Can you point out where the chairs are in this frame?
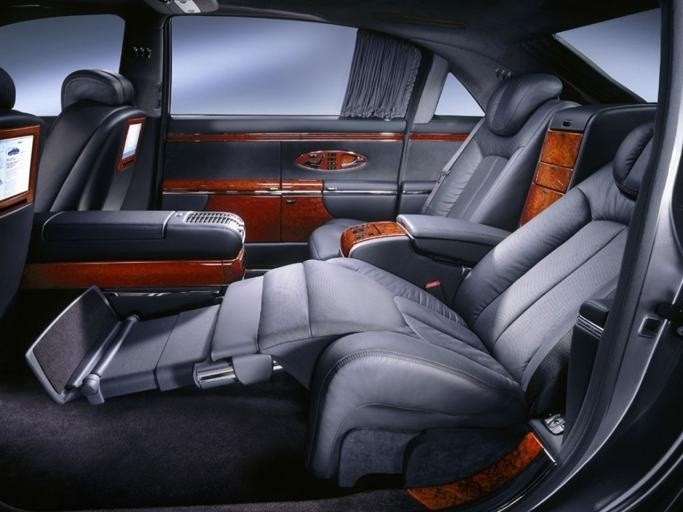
[301,70,585,263]
[21,115,658,486]
[33,66,151,214]
[2,69,46,313]
